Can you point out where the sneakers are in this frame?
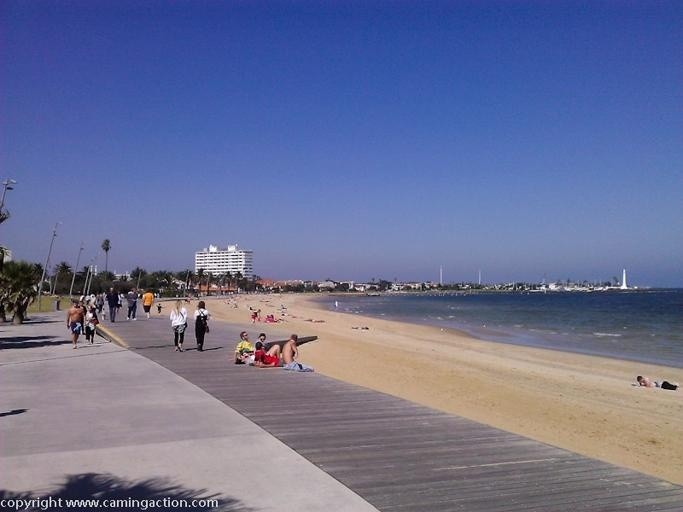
[176,344,184,352]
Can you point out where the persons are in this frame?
[630,374,679,391]
[255,332,280,359]
[169,300,189,351]
[255,341,281,368]
[282,334,314,372]
[223,295,287,324]
[235,353,256,364]
[194,300,209,352]
[66,286,163,350]
[233,331,254,363]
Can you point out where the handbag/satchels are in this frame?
[206,325,209,334]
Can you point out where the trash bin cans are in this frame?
[53,300,61,310]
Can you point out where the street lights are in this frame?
[38,221,63,311]
[70,240,98,296]
[1,178,17,210]
